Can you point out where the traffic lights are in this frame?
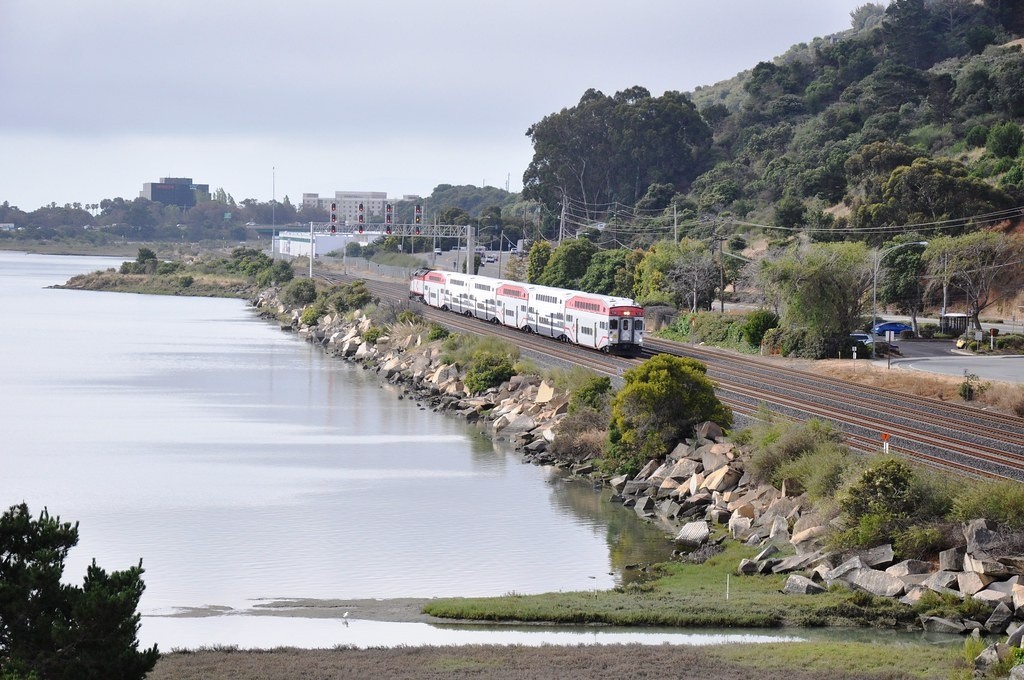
[358,214,364,224]
[358,224,363,233]
[331,213,336,223]
[415,226,421,235]
[414,213,421,224]
[385,203,391,213]
[414,205,422,214]
[385,214,391,223]
[331,202,336,211]
[386,225,392,234]
[331,224,336,234]
[358,203,364,211]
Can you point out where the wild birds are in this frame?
[343,612,349,620]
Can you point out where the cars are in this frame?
[475,244,498,267]
[510,248,518,255]
[434,247,442,255]
[849,333,873,345]
[869,322,913,337]
[866,341,901,358]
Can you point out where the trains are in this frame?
[408,269,646,359]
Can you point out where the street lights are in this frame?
[870,242,929,358]
[477,216,499,254]
